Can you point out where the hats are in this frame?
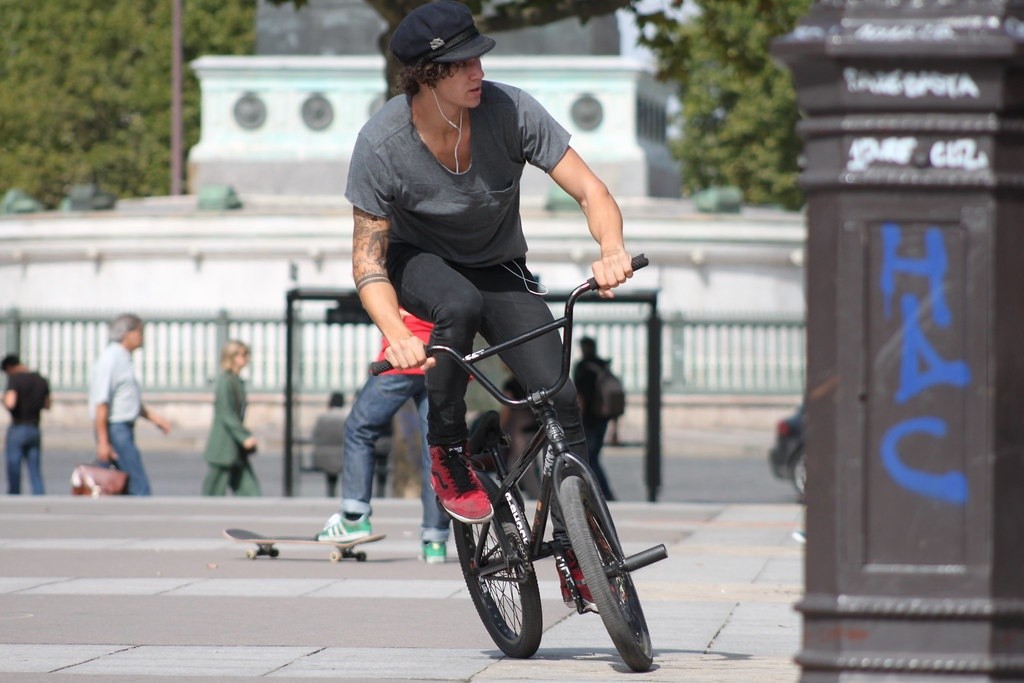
[390,1,497,62]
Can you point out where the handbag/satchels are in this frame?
[70,455,129,497]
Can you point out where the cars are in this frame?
[769,402,805,505]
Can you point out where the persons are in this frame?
[318,304,467,566]
[311,388,352,498]
[84,313,171,496]
[0,351,55,495]
[369,418,394,499]
[194,339,268,496]
[307,1,642,525]
[564,334,627,502]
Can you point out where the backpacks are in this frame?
[584,360,625,419]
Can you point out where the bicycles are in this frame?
[369,253,669,672]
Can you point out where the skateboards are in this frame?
[224,528,388,562]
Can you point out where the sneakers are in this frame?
[418,541,446,564]
[556,550,619,613]
[314,513,372,543]
[429,440,495,524]
